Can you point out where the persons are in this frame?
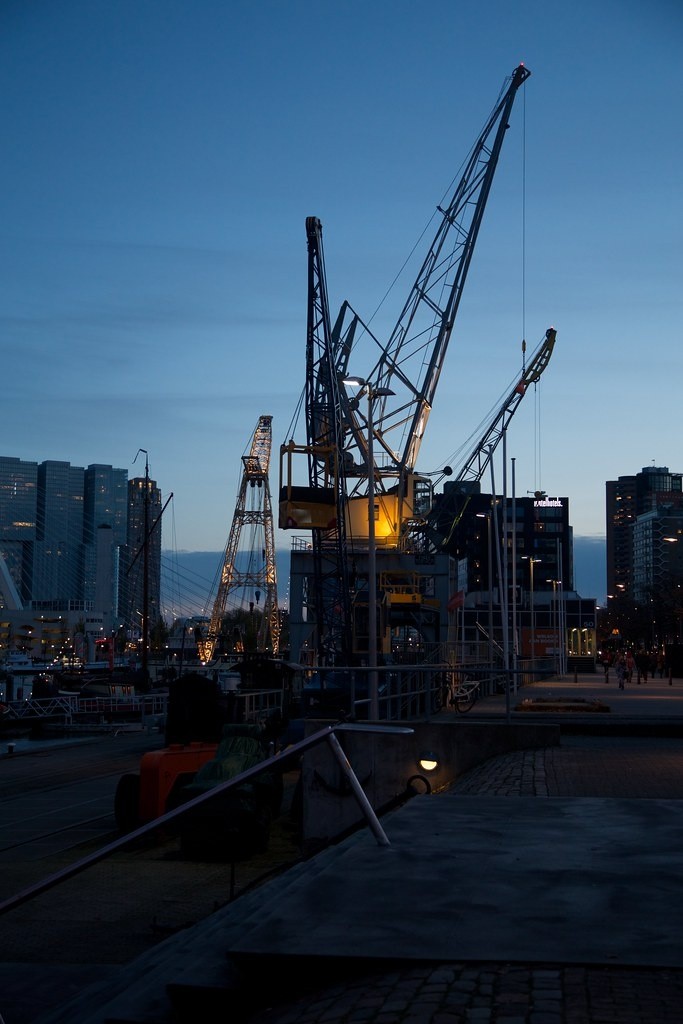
[600,648,665,691]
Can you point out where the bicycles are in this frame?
[432,658,481,712]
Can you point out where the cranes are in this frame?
[182,61,562,712]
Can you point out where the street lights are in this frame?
[546,579,562,660]
[520,555,543,658]
